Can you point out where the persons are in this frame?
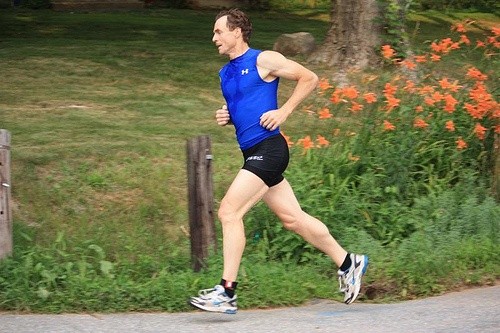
[187,7,369,314]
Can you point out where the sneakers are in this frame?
[187,283,237,314]
[337,252,368,304]
